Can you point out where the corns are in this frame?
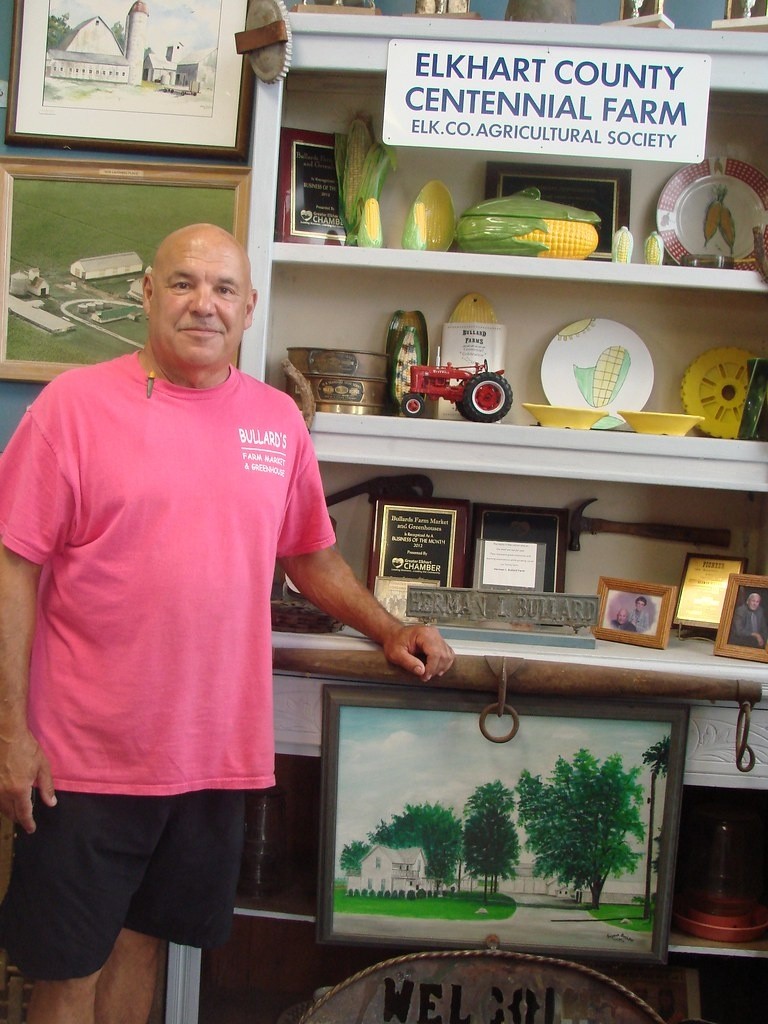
[390,326,422,417]
[332,119,665,266]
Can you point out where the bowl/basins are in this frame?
[617,409,705,436]
[522,403,609,430]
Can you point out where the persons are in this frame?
[628,596,650,632]
[0,220,454,1023]
[730,593,768,649]
[611,608,636,631]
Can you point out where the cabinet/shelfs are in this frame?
[162,12,768,1024]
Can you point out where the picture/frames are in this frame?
[374,576,440,626]
[713,573,768,663]
[1,155,265,384]
[589,576,679,649]
[484,161,631,261]
[4,0,254,160]
[318,685,688,967]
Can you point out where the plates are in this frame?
[540,318,655,429]
[656,154,768,271]
[384,309,430,417]
[416,179,456,252]
